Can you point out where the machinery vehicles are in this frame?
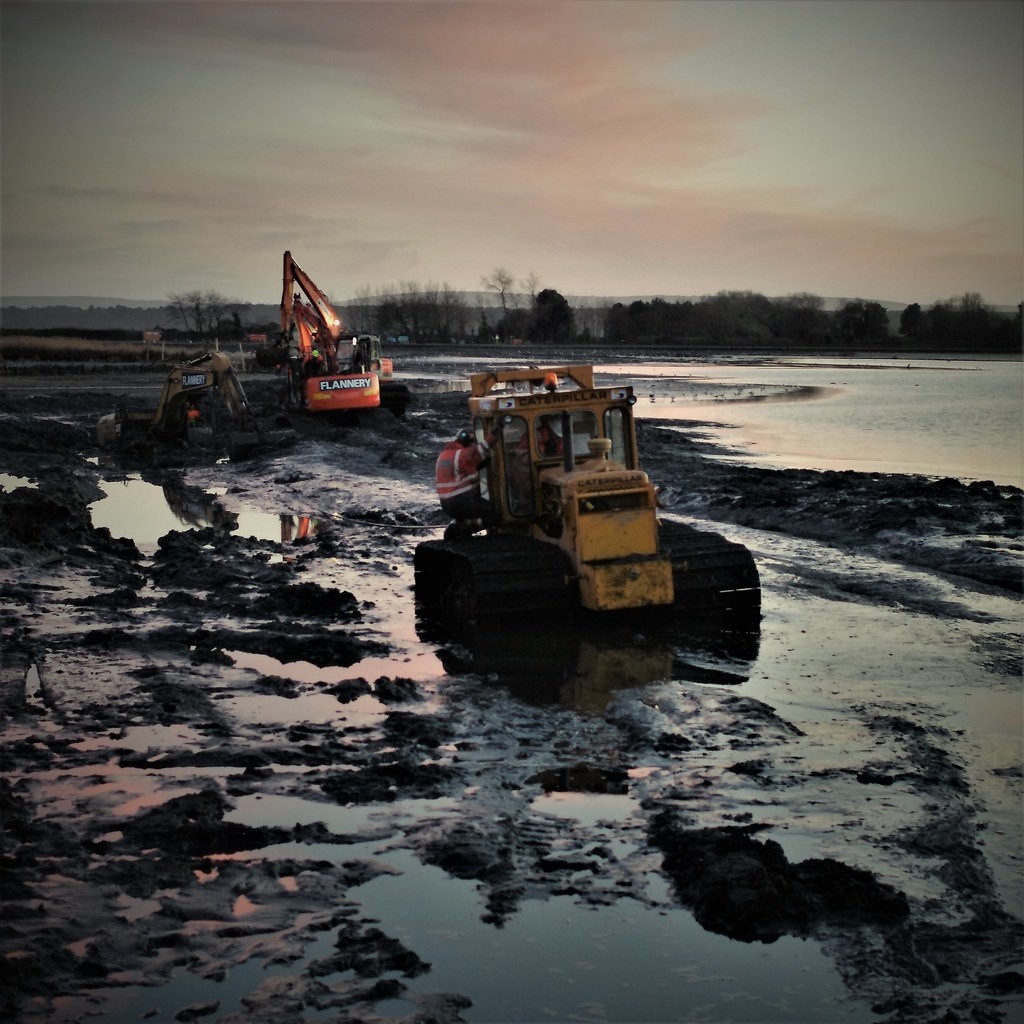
[96,351,279,466]
[255,250,411,440]
[413,364,762,706]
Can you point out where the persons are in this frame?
[295,351,306,407]
[436,424,503,534]
[495,334,499,345]
[188,404,205,427]
[312,349,325,377]
[510,418,564,513]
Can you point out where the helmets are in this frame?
[313,351,317,357]
[458,430,473,442]
